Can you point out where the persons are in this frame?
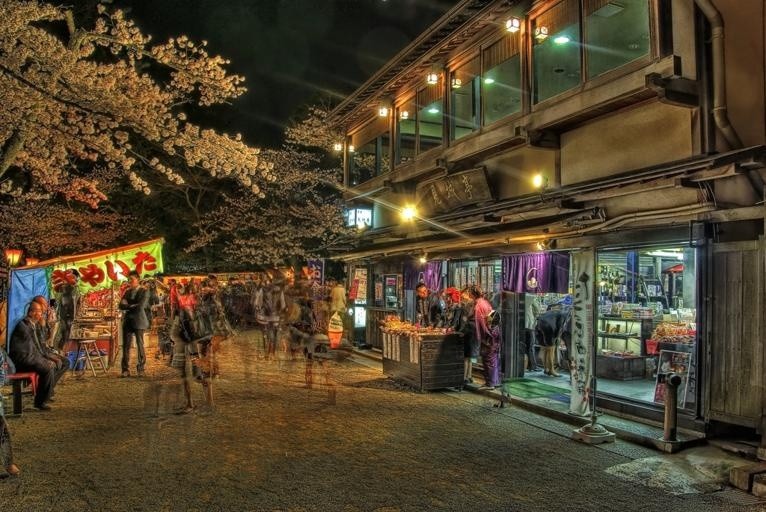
[0,412,20,477]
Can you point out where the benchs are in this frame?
[5,373,38,414]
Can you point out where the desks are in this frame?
[378,326,465,389]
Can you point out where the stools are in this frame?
[73,339,107,376]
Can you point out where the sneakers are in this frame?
[34,396,55,412]
[137,370,145,377]
[120,369,130,377]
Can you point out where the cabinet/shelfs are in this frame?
[641,274,671,315]
[596,316,653,380]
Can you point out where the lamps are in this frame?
[334,141,342,150]
[401,111,408,119]
[379,108,388,117]
[451,78,462,88]
[534,25,548,39]
[505,12,520,33]
[426,73,438,84]
[349,145,354,152]
[532,175,550,202]
